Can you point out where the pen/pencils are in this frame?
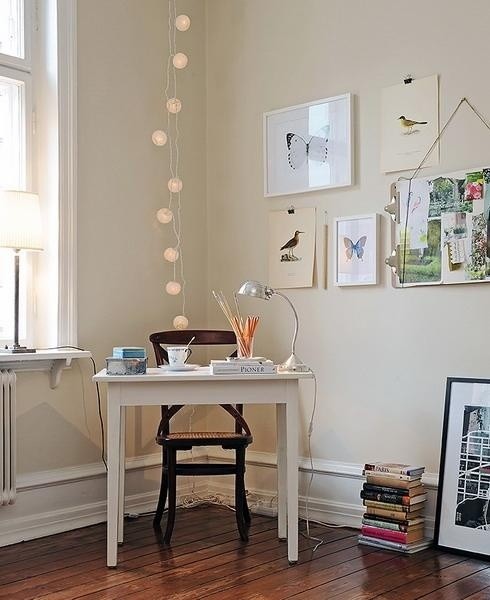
[231,315,259,358]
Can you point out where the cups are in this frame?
[237,337,254,359]
[167,347,192,367]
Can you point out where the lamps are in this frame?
[150,0,190,332]
[0,190,47,354]
[237,278,312,373]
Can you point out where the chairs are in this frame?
[149,327,255,546]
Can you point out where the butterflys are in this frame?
[344,236,367,262]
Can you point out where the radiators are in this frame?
[1,367,19,508]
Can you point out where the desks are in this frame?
[91,367,317,571]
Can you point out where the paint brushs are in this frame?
[212,289,250,358]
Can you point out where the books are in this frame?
[359,463,434,554]
[209,360,278,374]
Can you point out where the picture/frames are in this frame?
[260,92,355,198]
[430,376,490,563]
[332,212,383,288]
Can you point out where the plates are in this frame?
[160,365,199,371]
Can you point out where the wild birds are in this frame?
[279,230,305,260]
[397,116,428,135]
